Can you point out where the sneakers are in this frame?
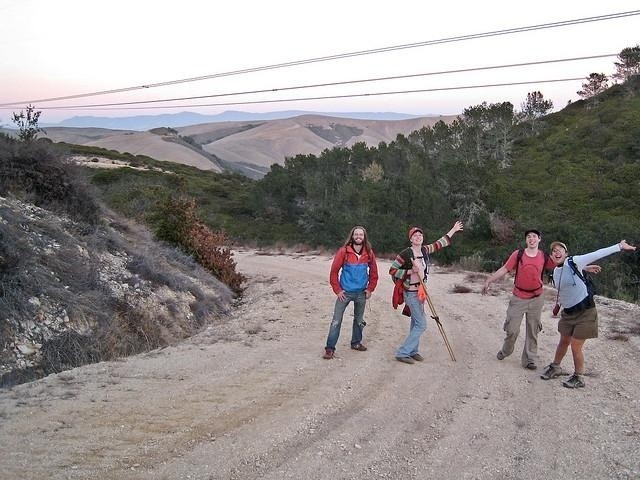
[540,362,563,381]
[563,372,585,388]
[496,349,509,360]
[394,356,416,365]
[323,345,336,360]
[350,343,368,351]
[411,353,425,361]
[526,360,537,370]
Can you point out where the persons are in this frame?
[540,240,636,388]
[323,226,378,359]
[389,221,463,363]
[481,229,602,368]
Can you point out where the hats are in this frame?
[408,226,424,239]
[524,228,542,237]
[550,240,568,253]
